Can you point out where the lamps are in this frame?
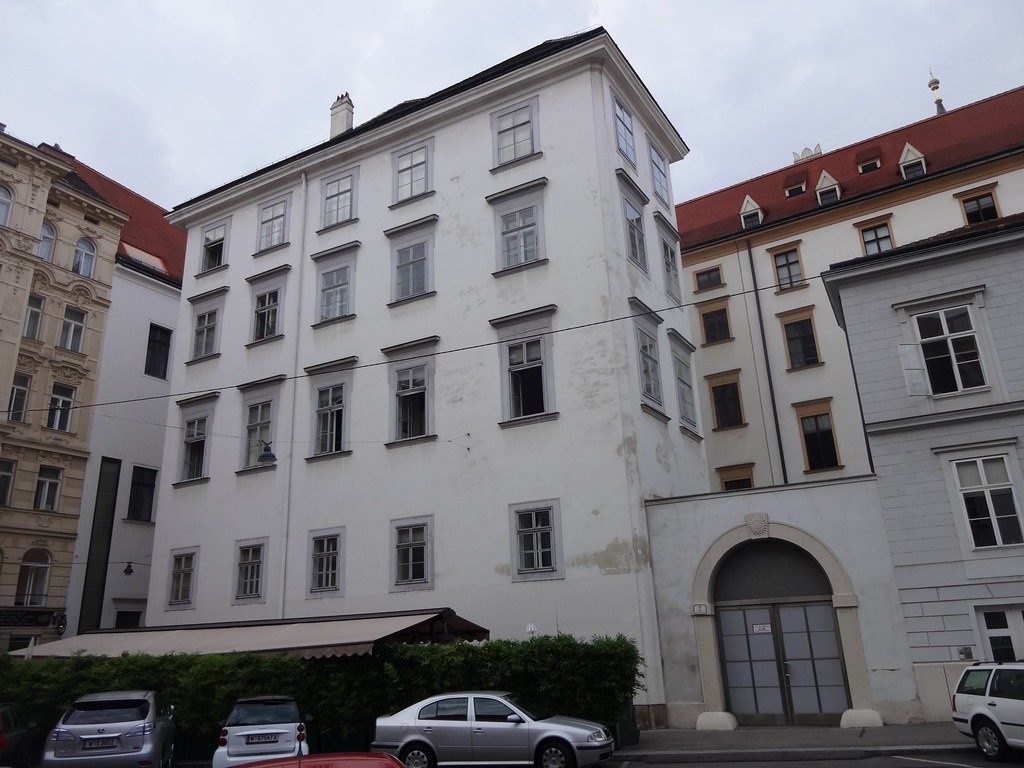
[256,442,278,466]
[526,622,536,638]
[122,563,134,575]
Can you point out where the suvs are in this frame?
[952,660,1024,762]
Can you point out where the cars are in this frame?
[370,690,616,768]
[39,689,176,768]
[227,751,405,768]
[212,696,309,768]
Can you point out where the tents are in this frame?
[5,605,490,660]
